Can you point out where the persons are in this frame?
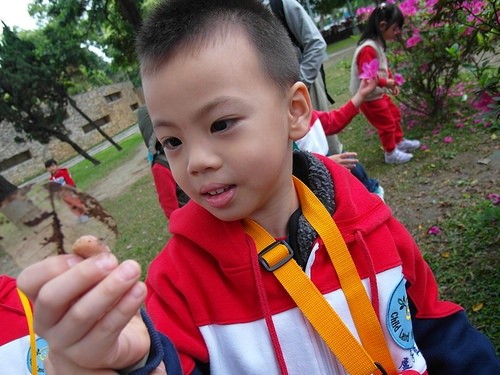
[45,160,76,187]
[1,274,49,374]
[17,0,500,375]
[136,105,191,220]
[295,60,385,203]
[348,4,421,164]
[262,0,344,157]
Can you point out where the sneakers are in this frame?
[395,139,420,152]
[374,185,384,202]
[384,149,413,164]
